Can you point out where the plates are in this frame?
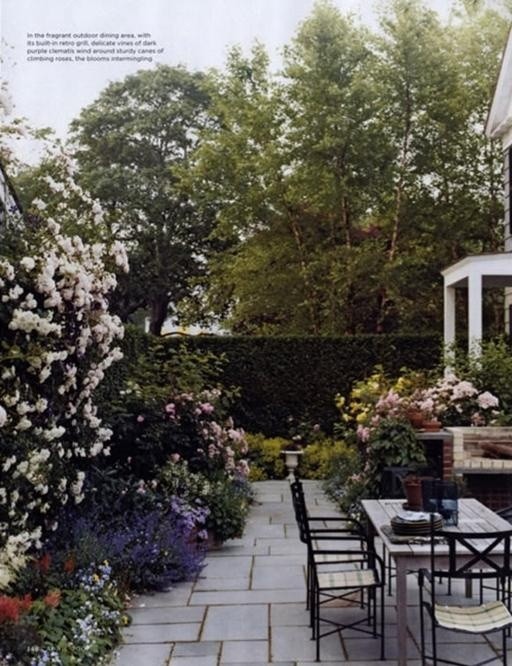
[390,512,443,537]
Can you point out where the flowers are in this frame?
[323,363,499,534]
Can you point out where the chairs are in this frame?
[288,469,511,664]
